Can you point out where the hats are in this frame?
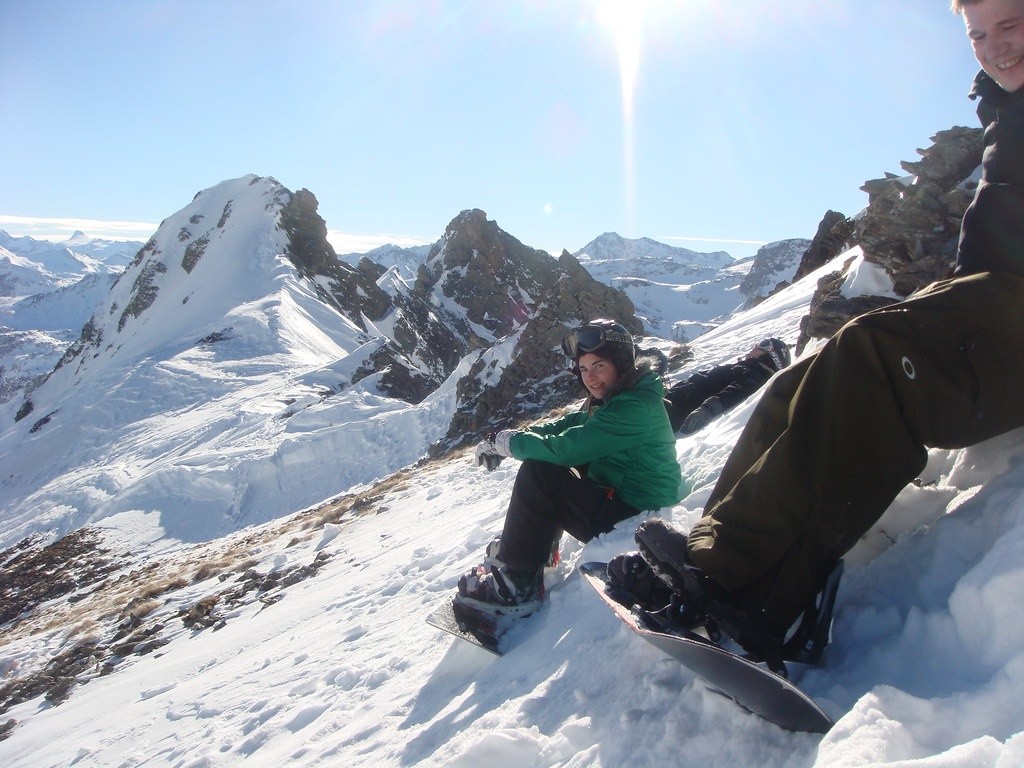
[761,338,791,370]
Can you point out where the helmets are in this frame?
[564,319,635,373]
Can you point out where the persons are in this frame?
[604,0,1024,669]
[457,319,681,617]
[664,339,790,434]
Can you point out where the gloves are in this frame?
[475,426,518,471]
[680,405,712,435]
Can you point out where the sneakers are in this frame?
[457,564,546,615]
[635,517,827,658]
[484,529,560,568]
[606,550,723,644]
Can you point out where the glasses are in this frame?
[757,339,772,352]
[561,324,607,359]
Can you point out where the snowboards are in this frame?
[422,535,576,656]
[580,561,835,734]
